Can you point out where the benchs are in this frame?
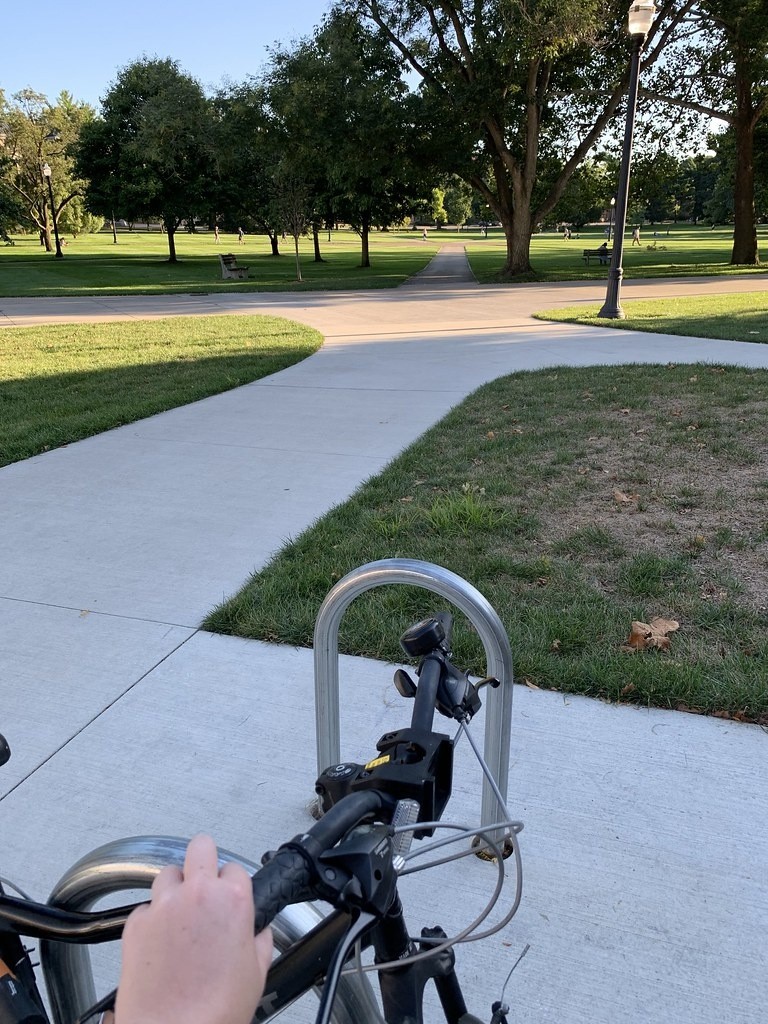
[220,253,248,282]
[581,249,613,267]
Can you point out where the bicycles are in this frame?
[1,610,530,1024]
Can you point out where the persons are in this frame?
[481,226,485,236]
[666,225,670,235]
[711,224,715,231]
[412,225,417,231]
[604,225,613,238]
[280,231,289,244]
[238,227,245,245]
[214,226,222,245]
[564,226,569,241]
[381,226,389,232]
[632,226,642,246]
[114,832,272,1024]
[423,228,427,240]
[60,238,69,246]
[599,243,608,265]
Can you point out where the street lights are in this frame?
[485,204,489,238]
[597,0,656,319]
[608,198,616,241]
[44,163,64,257]
